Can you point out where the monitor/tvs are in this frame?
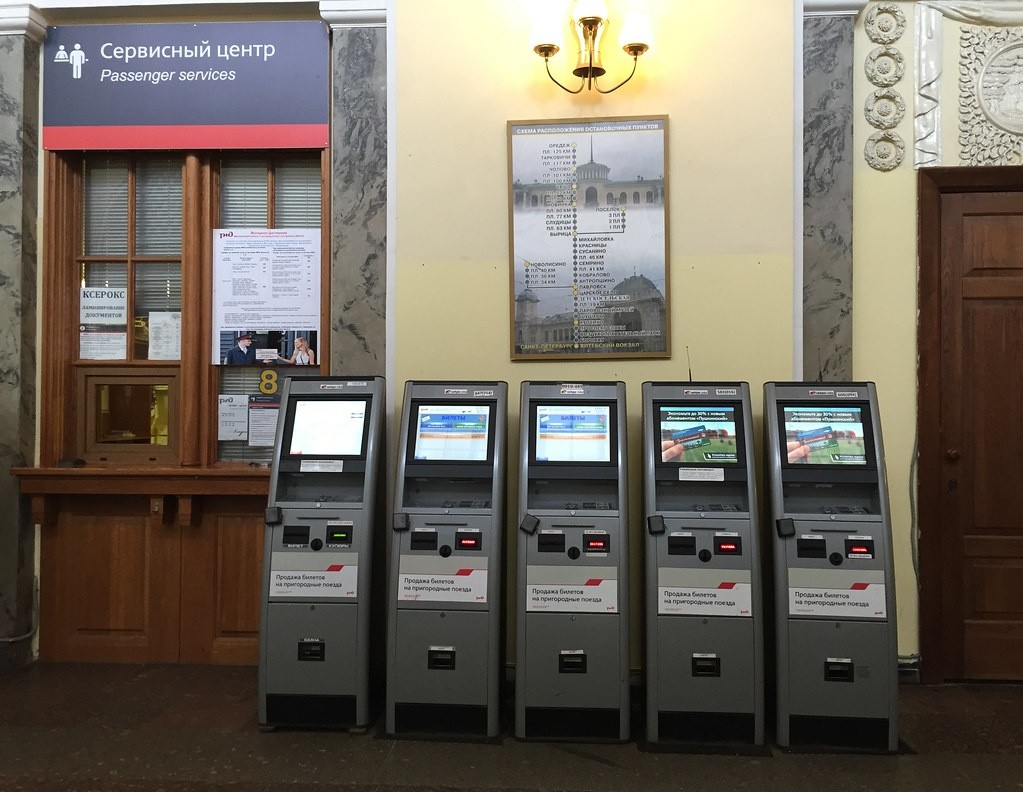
[281,398,372,461]
[528,401,618,467]
[652,402,747,469]
[405,401,495,465]
[777,403,877,471]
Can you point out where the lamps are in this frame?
[533,2,652,94]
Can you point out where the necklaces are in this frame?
[298,349,302,351]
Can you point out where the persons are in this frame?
[662,441,683,462]
[787,442,810,463]
[224,334,273,365]
[274,337,314,365]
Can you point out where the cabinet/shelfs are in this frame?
[10,20,335,670]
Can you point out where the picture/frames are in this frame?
[506,115,674,361]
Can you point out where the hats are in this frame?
[234,334,252,341]
